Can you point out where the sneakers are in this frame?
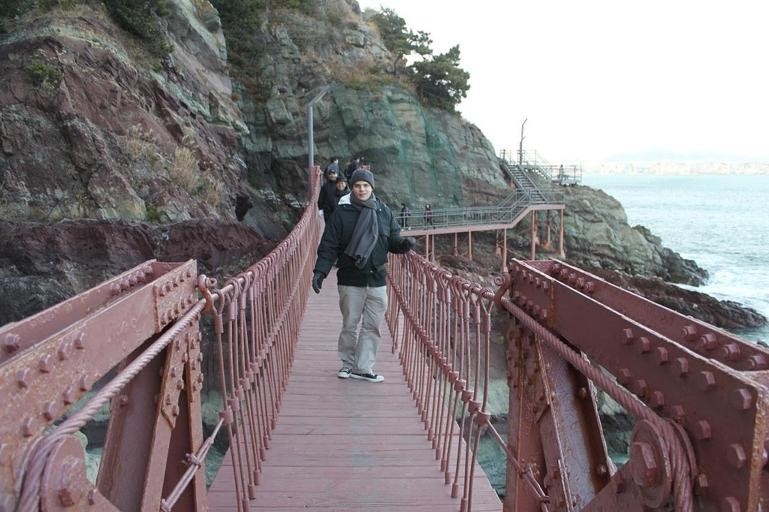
[337,366,385,383]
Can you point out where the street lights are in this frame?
[306,85,331,201]
[520,118,527,166]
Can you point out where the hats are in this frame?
[326,160,348,182]
[348,168,375,190]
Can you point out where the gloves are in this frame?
[406,236,416,251]
[311,271,325,293]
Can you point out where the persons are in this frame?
[318,156,370,225]
[399,203,411,231]
[557,163,566,186]
[312,168,415,382]
[424,204,435,230]
